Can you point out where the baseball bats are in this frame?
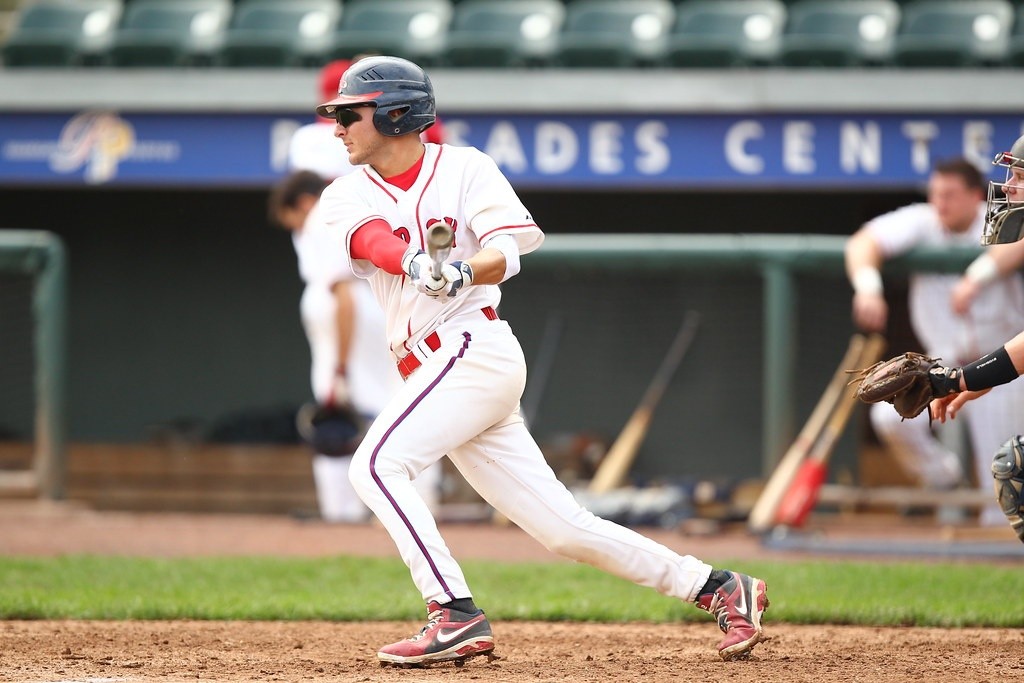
[589,315,697,496]
[491,321,564,529]
[748,334,884,532]
[426,223,454,279]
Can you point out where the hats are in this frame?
[296,401,368,457]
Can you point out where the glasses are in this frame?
[335,107,376,129]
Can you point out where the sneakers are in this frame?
[377,601,496,667]
[696,570,767,660]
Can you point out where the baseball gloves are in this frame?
[857,352,947,420]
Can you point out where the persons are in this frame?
[319,56,772,668]
[840,129,1024,540]
[266,169,440,524]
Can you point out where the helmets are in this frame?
[315,56,436,137]
[979,133,1024,244]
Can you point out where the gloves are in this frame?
[401,245,473,302]
